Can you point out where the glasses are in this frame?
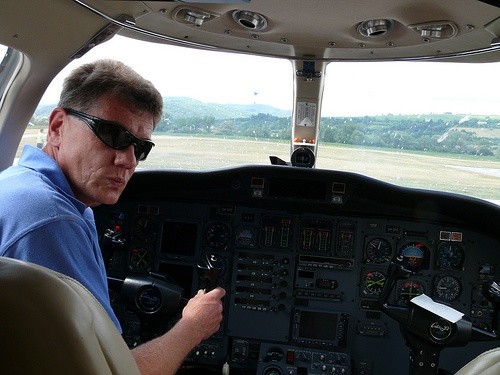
[63,107,155,162]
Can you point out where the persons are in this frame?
[1,57,228,374]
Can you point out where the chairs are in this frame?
[0,256,141,375]
